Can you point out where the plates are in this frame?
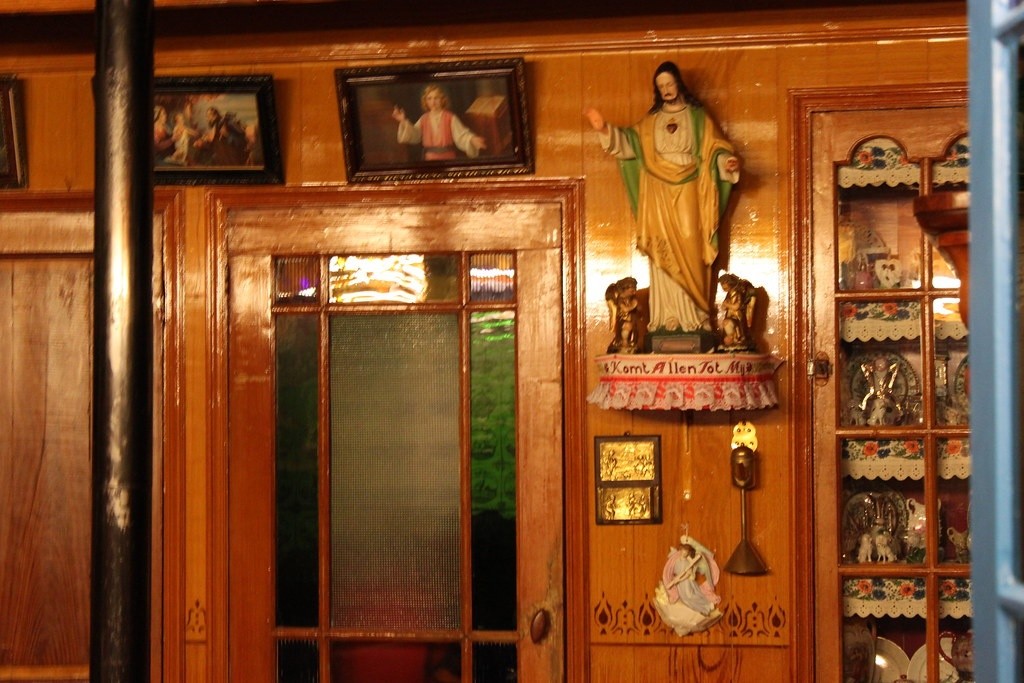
[954,354,969,413]
[872,637,910,683]
[841,481,909,559]
[906,637,958,683]
[841,349,919,404]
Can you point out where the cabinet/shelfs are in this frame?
[789,79,978,683]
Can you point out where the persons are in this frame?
[717,274,757,345]
[605,277,638,353]
[662,542,717,617]
[586,62,741,335]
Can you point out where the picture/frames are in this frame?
[148,71,287,186]
[0,78,26,190]
[334,56,536,182]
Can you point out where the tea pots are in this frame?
[938,631,974,683]
